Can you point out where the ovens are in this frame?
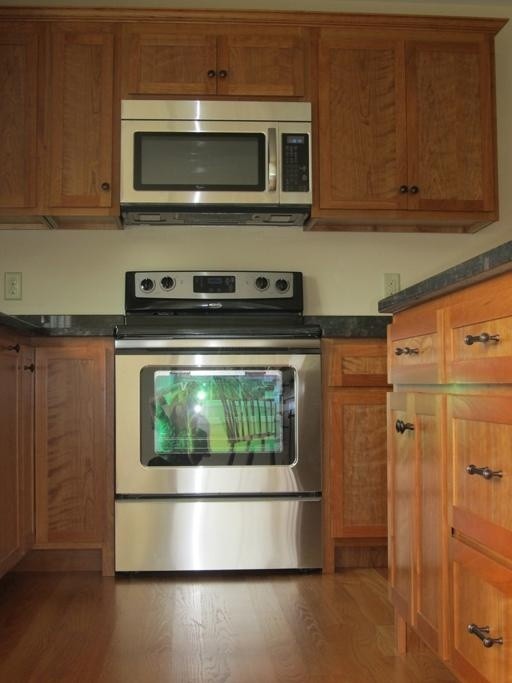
[111,352,323,578]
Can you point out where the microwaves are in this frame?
[118,99,311,207]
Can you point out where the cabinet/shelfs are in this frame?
[384,271,511,682]
[0,328,386,583]
[2,5,501,235]
